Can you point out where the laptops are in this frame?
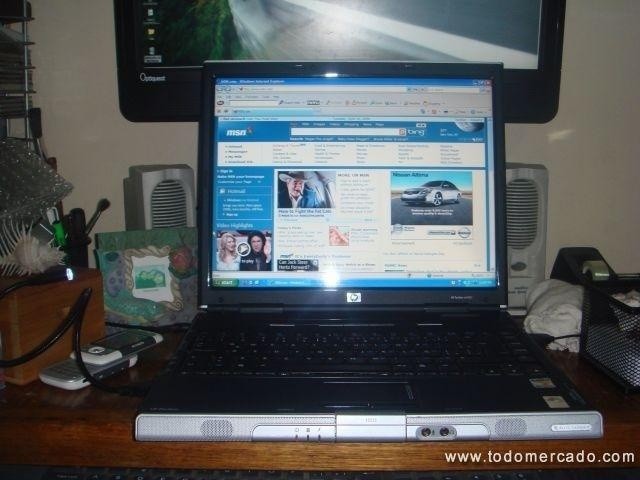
[133,59,604,443]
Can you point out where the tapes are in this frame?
[580,260,610,282]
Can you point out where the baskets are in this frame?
[576,275,640,394]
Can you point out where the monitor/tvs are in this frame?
[114,1,567,123]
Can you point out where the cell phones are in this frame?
[38,328,163,391]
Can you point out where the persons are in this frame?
[216,231,271,271]
[278,171,324,207]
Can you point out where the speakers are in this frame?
[123,164,197,231]
[505,162,547,309]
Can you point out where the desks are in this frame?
[1,319,637,471]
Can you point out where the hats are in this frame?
[278,171,314,184]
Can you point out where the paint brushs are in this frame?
[29,108,110,248]
